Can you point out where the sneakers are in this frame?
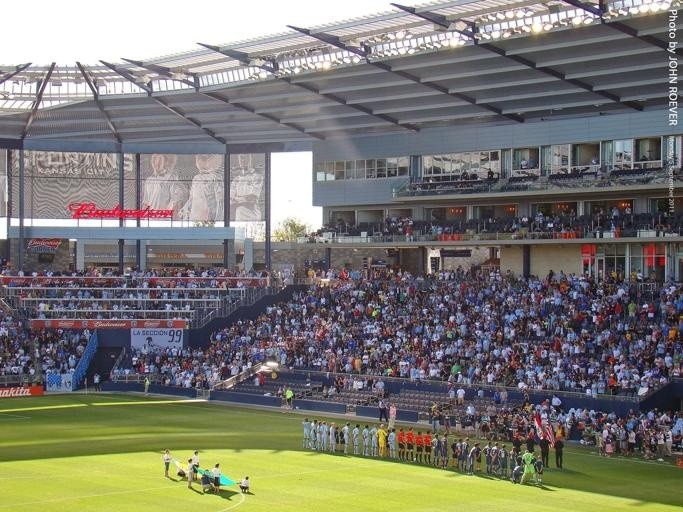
[302,442,545,486]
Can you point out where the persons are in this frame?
[178,154,223,220]
[143,153,189,210]
[0,156,683,496]
[230,154,264,220]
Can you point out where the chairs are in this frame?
[0,173,682,464]
